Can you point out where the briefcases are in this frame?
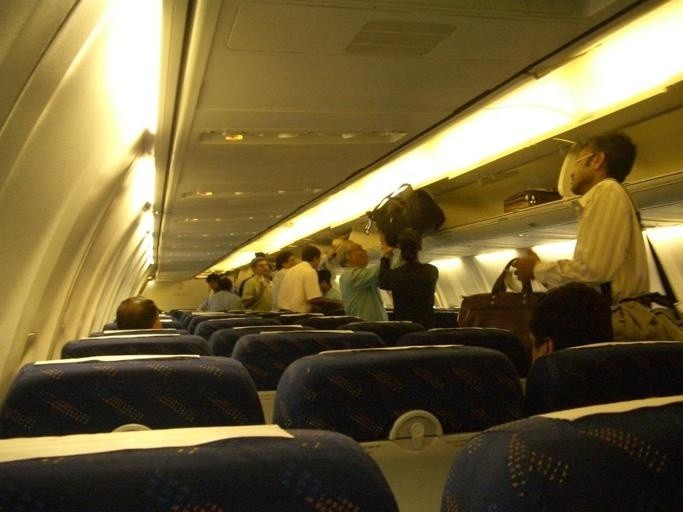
[503,190,561,212]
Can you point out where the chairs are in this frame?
[0,306,683,512]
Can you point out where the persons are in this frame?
[512,130,650,305]
[117,296,162,328]
[526,281,614,363]
[336,240,394,321]
[377,226,439,331]
[198,244,341,314]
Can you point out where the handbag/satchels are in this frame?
[458,291,544,336]
[612,302,682,341]
[371,190,445,247]
